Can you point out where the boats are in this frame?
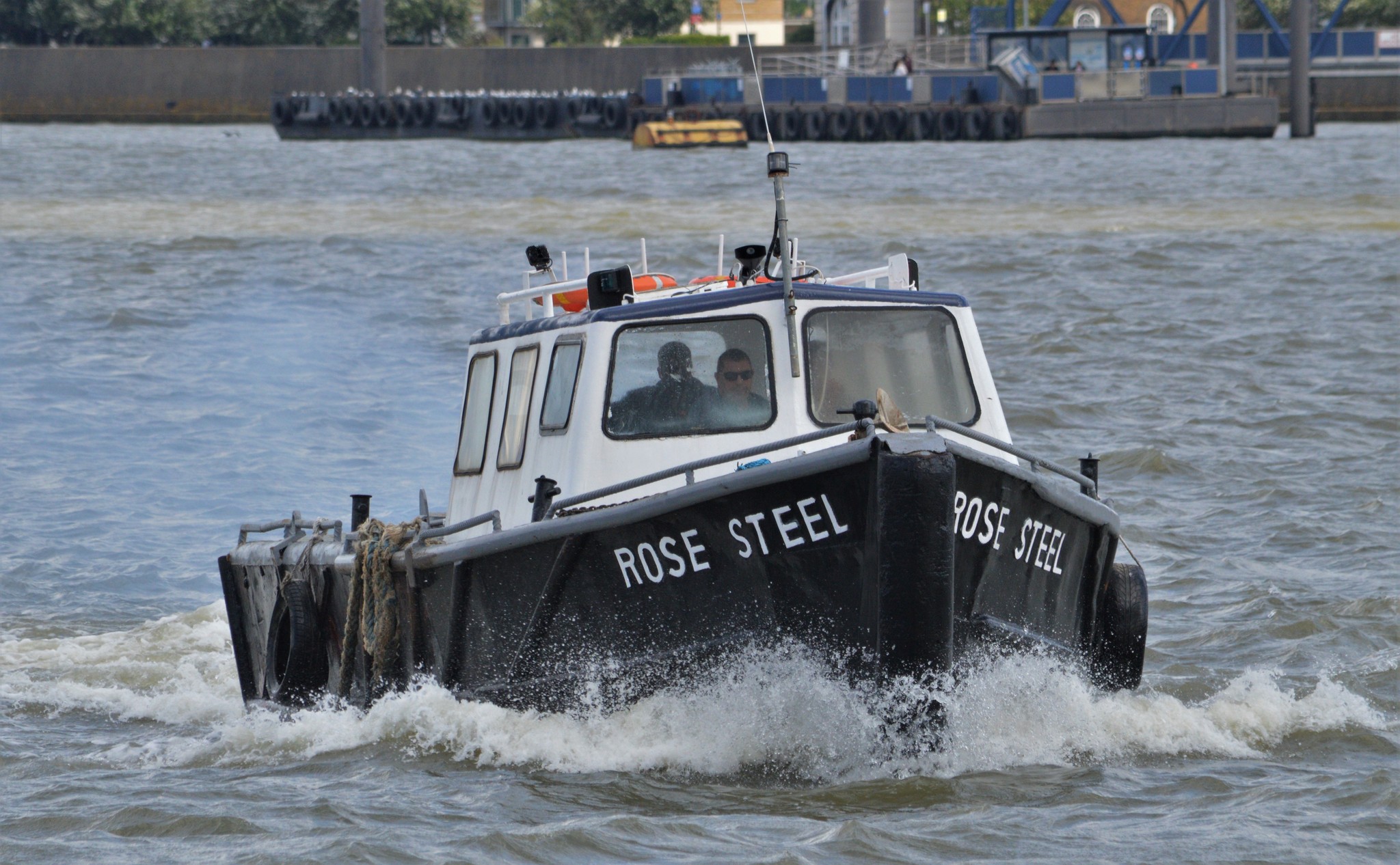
[217,0,1150,755]
[631,111,748,149]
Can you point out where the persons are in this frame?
[687,349,770,429]
[623,341,716,431]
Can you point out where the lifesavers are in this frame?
[532,272,678,313]
[688,275,809,288]
[269,96,1018,143]
[264,580,317,709]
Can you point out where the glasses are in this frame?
[720,369,754,381]
